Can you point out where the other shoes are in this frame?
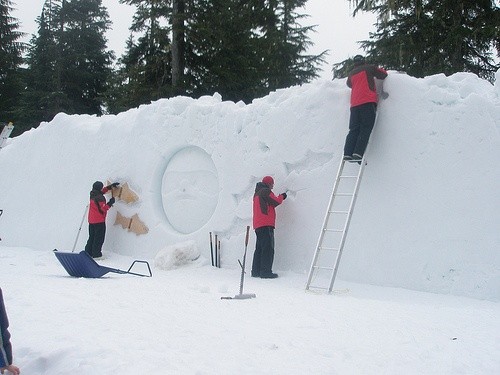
[252,272,278,278]
[343,152,368,166]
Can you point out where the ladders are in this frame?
[305,111,379,293]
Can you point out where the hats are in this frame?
[93,181,103,190]
[262,175,274,185]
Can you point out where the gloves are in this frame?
[107,182,120,190]
[282,193,287,200]
[107,197,115,207]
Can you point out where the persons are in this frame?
[85,181,120,258]
[0,288,19,375]
[343,55,388,165]
[251,176,287,279]
